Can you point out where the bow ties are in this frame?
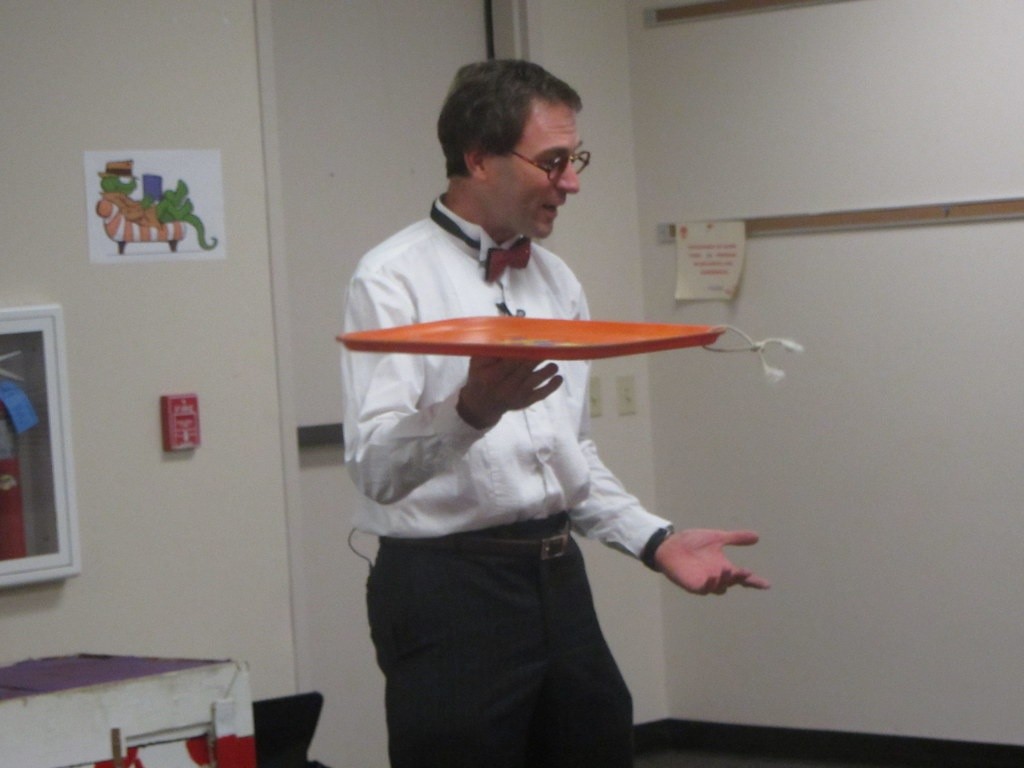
[431,199,531,282]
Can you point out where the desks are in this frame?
[0,655,254,768]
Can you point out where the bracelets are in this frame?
[642,526,675,572]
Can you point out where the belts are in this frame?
[379,513,571,560]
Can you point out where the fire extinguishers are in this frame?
[0,350,28,561]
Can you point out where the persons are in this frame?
[340,61,770,768]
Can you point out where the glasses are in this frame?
[509,150,590,181]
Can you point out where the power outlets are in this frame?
[614,374,638,417]
